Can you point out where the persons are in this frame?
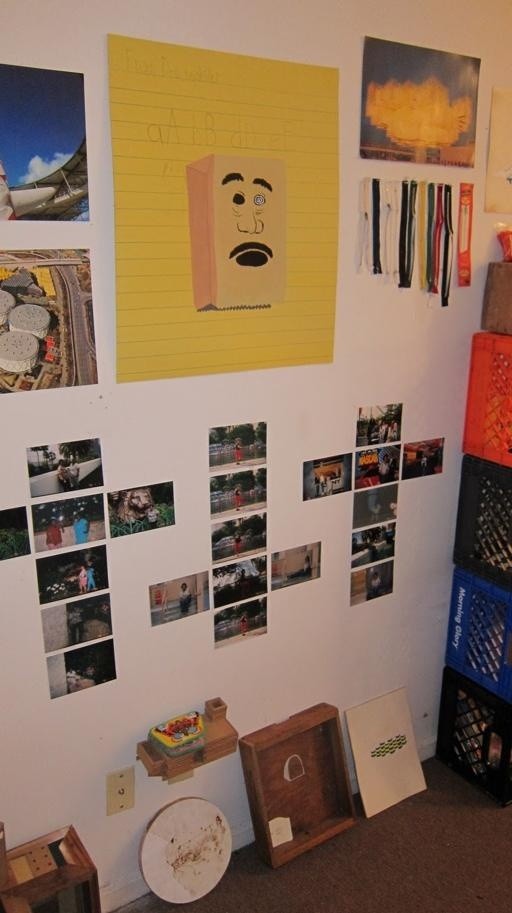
[371,572,381,595]
[352,536,377,555]
[143,504,161,528]
[56,457,79,492]
[46,517,62,550]
[66,667,77,678]
[68,606,84,643]
[71,512,89,543]
[178,582,192,614]
[233,437,250,636]
[315,470,333,496]
[367,416,398,484]
[288,555,309,579]
[78,565,98,594]
